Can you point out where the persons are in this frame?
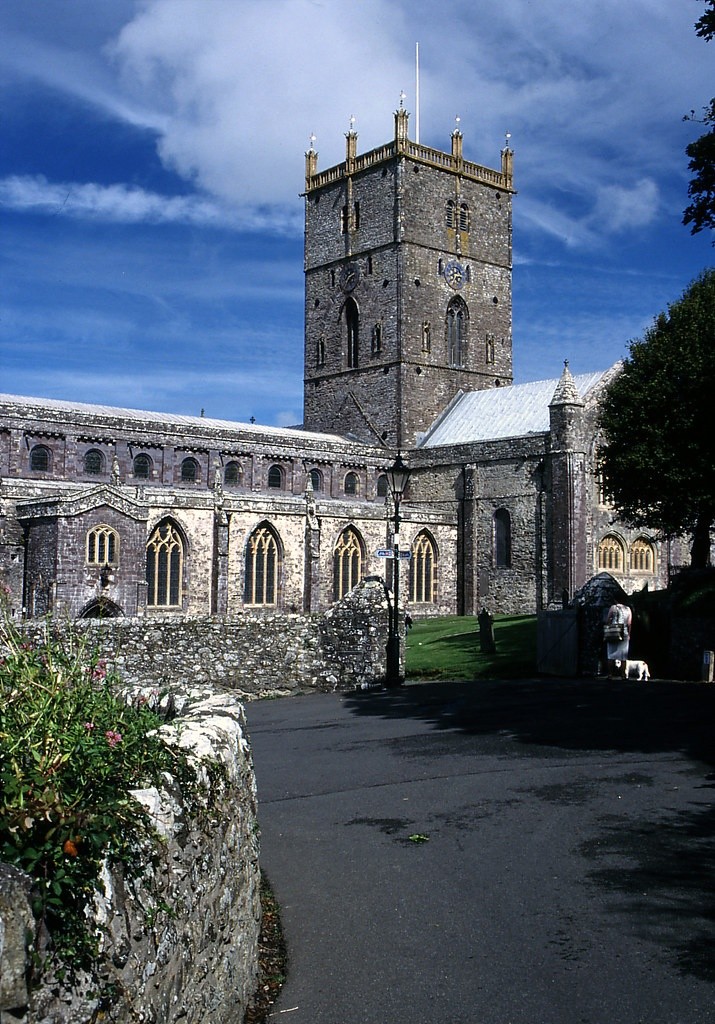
[605,591,632,681]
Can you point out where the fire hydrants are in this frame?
[478,609,497,651]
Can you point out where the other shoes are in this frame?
[608,675,612,680]
[621,674,626,680]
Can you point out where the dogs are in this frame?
[615,659,650,681]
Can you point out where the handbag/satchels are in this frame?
[602,606,624,642]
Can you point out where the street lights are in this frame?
[385,454,412,688]
